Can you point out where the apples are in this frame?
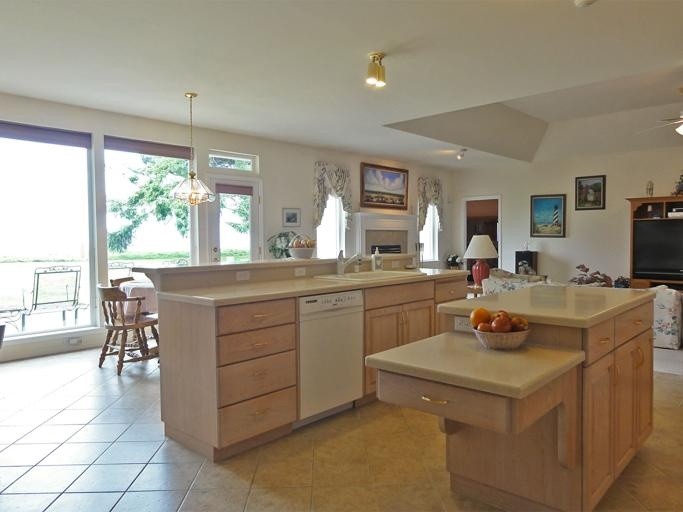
[470,306,529,334]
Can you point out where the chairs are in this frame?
[98,275,159,375]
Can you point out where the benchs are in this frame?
[0,262,138,333]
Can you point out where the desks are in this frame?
[467,284,482,297]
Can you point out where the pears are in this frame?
[290,238,316,248]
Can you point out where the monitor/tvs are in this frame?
[632,218,683,281]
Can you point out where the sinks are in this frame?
[313,270,427,281]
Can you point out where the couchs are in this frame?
[480,268,682,349]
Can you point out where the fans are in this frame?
[634,79,683,139]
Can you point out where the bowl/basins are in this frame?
[471,327,530,350]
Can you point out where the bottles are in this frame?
[645,180,653,197]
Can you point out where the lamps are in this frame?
[463,236,499,288]
[169,92,215,206]
[675,88,683,135]
[366,50,385,87]
[457,149,467,159]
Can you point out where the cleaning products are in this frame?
[372,247,383,272]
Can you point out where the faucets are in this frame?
[336,250,360,275]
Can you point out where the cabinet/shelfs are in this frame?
[630,277,683,294]
[627,196,683,290]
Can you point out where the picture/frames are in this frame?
[575,175,605,210]
[281,207,301,228]
[360,162,409,210]
[529,194,566,237]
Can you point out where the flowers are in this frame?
[447,254,463,266]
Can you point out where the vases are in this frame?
[450,266,459,270]
